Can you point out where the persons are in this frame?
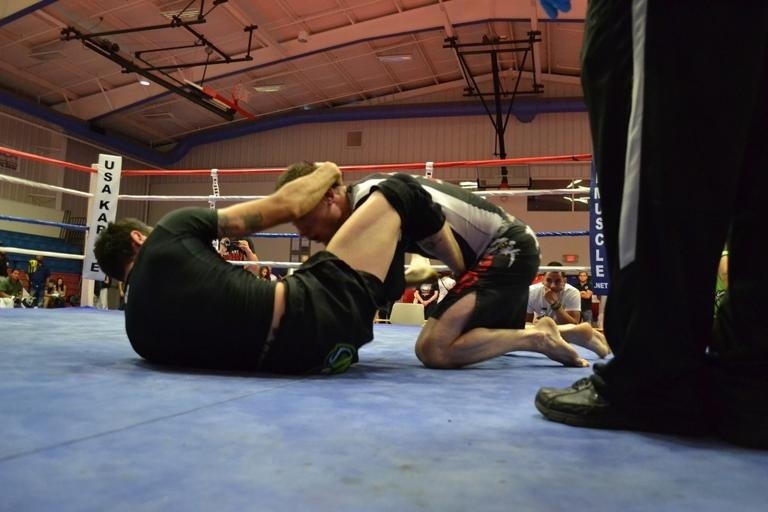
[535,0,768,435]
[0,256,67,308]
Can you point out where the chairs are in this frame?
[375,301,428,327]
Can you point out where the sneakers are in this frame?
[534,377,659,433]
